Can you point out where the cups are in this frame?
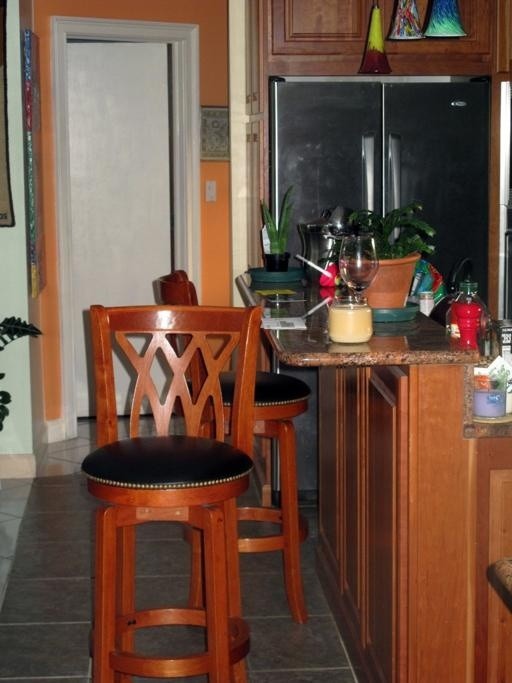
[328,296,373,343]
[338,235,379,302]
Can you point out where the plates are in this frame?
[243,266,303,282]
[371,301,421,321]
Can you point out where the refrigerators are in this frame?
[269,71,491,509]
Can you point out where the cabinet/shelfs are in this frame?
[237,275,512,683]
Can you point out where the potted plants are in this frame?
[336,204,435,309]
[262,188,295,272]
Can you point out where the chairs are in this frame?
[159,271,310,624]
[78,304,265,683]
